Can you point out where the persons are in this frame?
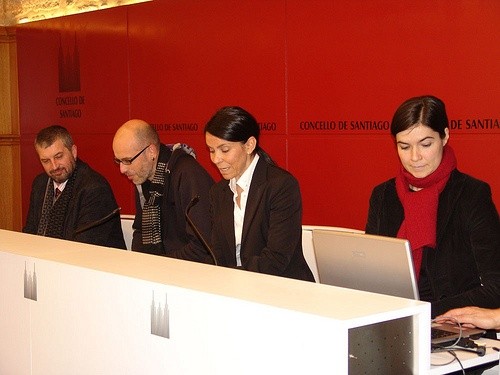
[202,106,316,283]
[22,125,127,250]
[112,119,216,261]
[365,95,500,331]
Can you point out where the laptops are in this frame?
[312,228,487,347]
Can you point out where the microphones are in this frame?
[185,194,218,267]
[72,208,121,240]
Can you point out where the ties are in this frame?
[54,188,61,199]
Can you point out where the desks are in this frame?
[430,338,500,375]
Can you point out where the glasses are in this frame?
[111,147,147,165]
